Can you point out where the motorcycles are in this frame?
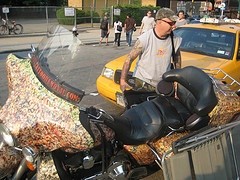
[0,25,240,180]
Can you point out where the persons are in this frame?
[176,10,187,26]
[99,16,110,46]
[120,8,182,109]
[124,13,136,46]
[113,17,122,46]
[140,10,156,34]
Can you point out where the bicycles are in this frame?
[0,18,24,35]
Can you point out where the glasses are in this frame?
[158,19,176,26]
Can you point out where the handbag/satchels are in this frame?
[117,25,122,31]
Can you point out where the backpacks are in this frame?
[100,18,108,30]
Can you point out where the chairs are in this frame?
[186,32,207,48]
[105,65,218,145]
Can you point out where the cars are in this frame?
[96,24,240,110]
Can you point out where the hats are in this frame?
[155,8,180,21]
